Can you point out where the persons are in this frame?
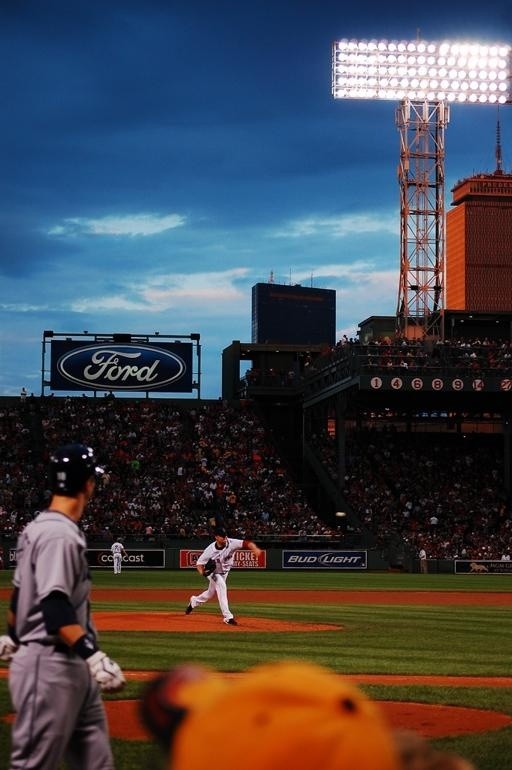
[1,327,512,577]
[0,441,127,769]
[138,659,475,770]
[185,528,265,627]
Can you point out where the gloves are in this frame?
[0,635,17,662]
[84,649,127,695]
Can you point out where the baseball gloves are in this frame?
[202,558,216,577]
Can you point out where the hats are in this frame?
[214,526,227,537]
[170,662,402,770]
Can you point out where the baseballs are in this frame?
[335,512,346,517]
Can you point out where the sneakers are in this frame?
[223,617,239,625]
[114,572,121,576]
[185,602,193,614]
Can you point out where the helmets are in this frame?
[45,443,106,489]
